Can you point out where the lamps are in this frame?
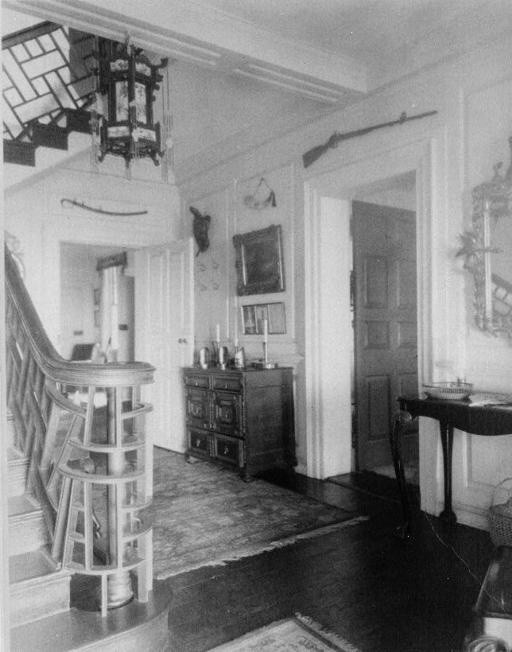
[90,39,169,170]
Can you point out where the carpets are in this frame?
[63,445,370,582]
[203,612,361,652]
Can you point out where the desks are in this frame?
[391,391,512,539]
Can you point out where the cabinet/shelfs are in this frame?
[182,367,298,483]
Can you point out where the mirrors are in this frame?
[470,160,512,338]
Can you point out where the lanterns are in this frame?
[89,35,165,166]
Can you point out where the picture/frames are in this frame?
[243,301,287,334]
[234,224,284,296]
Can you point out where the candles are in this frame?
[216,323,221,344]
[264,319,267,342]
[234,318,238,346]
